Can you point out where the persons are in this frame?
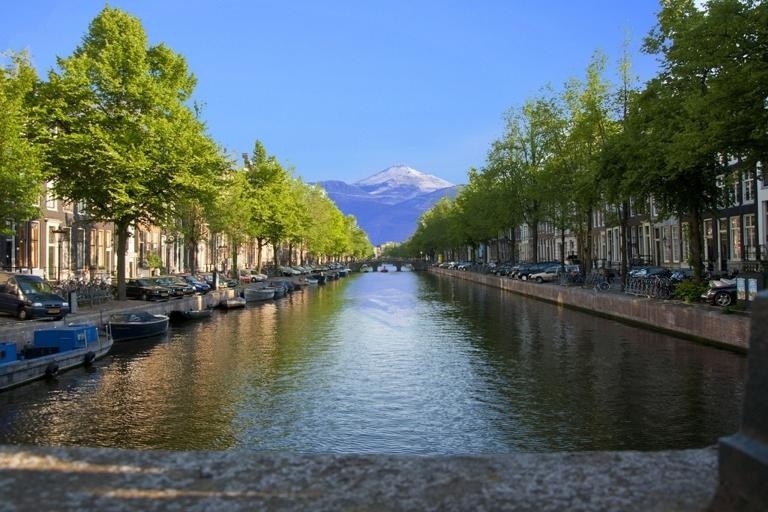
[705,259,717,280]
[80,264,90,289]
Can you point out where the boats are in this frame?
[220,297,246,309]
[244,285,274,301]
[274,269,352,298]
[170,310,213,321]
[0,323,114,395]
[103,312,170,342]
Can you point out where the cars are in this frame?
[263,262,343,276]
[433,260,477,271]
[701,288,737,306]
[125,269,268,301]
[672,269,699,280]
[487,262,580,283]
[628,265,669,274]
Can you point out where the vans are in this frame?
[0,273,70,320]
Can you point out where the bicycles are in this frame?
[592,274,674,299]
[45,274,118,307]
